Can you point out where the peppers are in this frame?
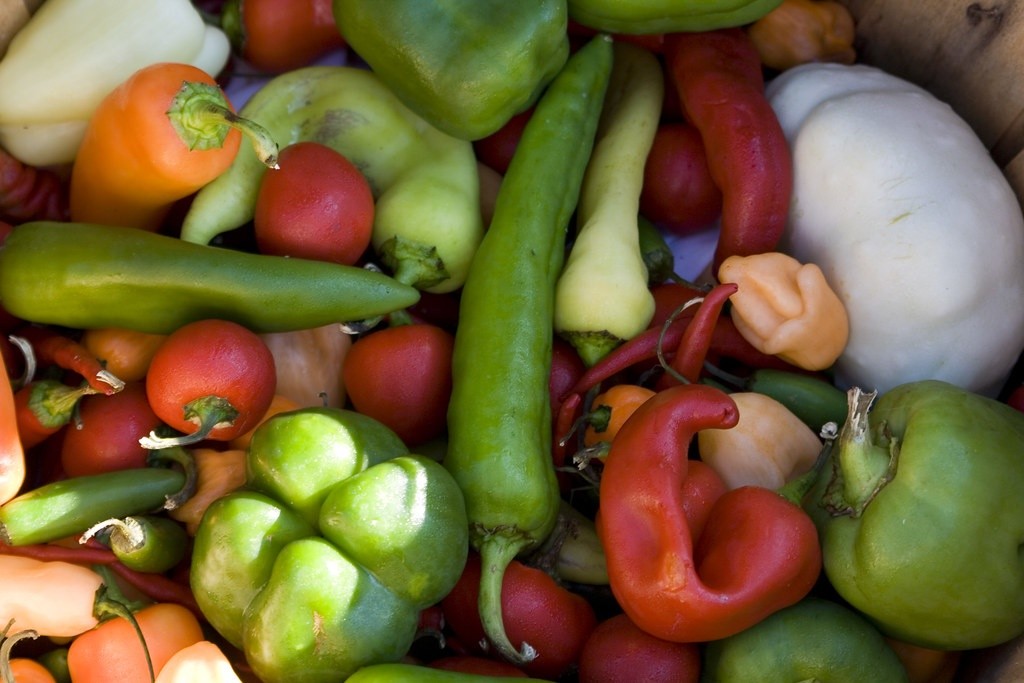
[0,0,1024,683]
